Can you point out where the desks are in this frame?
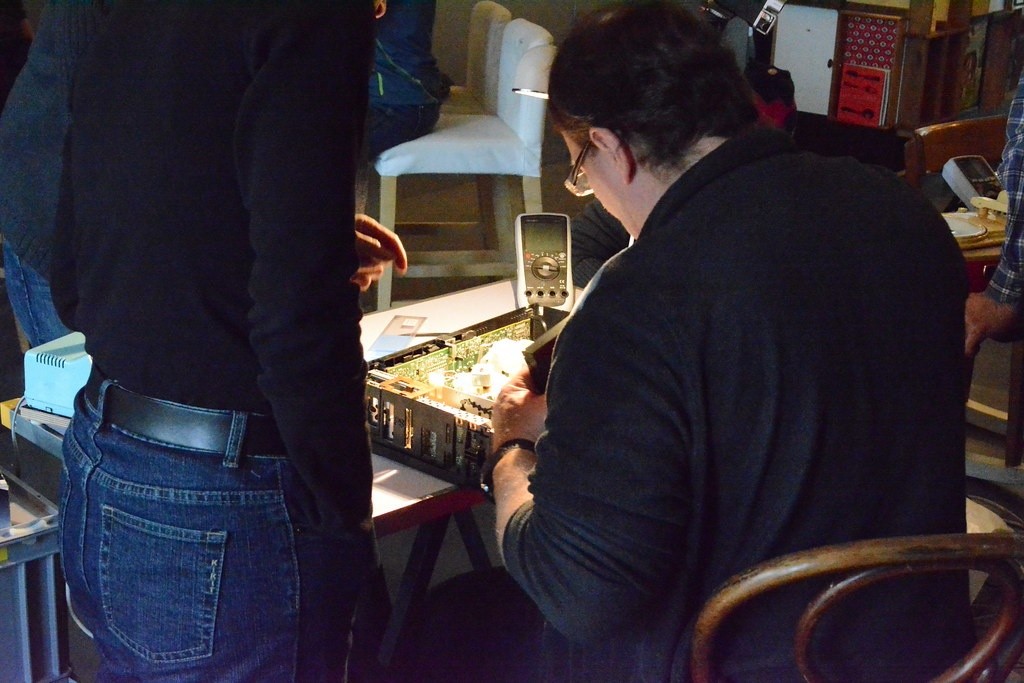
[0,280,590,683]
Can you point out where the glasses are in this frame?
[564,139,594,197]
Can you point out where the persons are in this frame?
[571,198,633,288]
[1,1,87,347]
[46,1,406,683]
[352,0,445,170]
[476,1,980,683]
[962,73,1023,358]
[744,62,797,131]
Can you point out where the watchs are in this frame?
[479,439,536,503]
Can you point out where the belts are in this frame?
[84,363,288,459]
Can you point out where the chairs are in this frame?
[368,18,557,313]
[689,531,1024,682]
[353,0,511,283]
